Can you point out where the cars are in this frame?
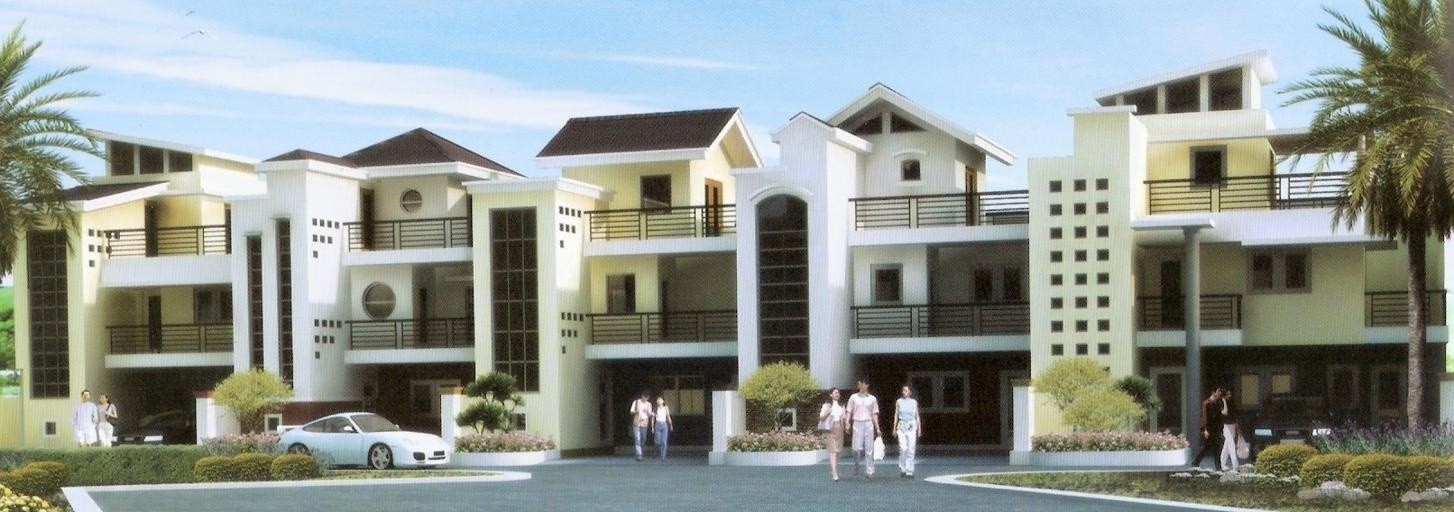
[111,409,196,446]
[1235,394,1340,465]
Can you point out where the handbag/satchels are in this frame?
[817,402,833,432]
[629,424,634,437]
[874,436,886,461]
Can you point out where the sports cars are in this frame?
[275,411,451,469]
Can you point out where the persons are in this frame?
[891,384,921,478]
[93,391,117,446]
[818,387,847,481]
[1217,384,1241,473]
[844,377,882,479]
[629,389,652,461]
[1188,383,1224,472]
[649,394,674,461]
[69,389,98,447]
